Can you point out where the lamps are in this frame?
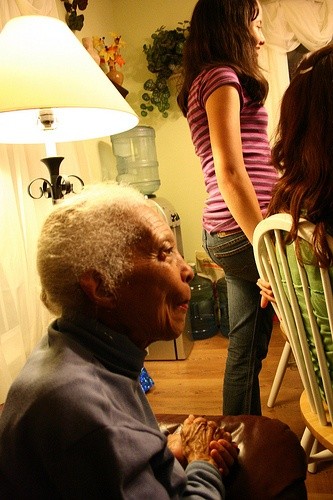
[0,15,139,202]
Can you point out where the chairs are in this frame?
[249,214,333,475]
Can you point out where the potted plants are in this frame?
[140,22,192,117]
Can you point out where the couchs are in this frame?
[155,413,307,500]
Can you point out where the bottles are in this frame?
[215,276,230,338]
[111,126,160,196]
[186,263,219,340]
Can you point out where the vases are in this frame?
[98,56,124,84]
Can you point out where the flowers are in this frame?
[92,29,126,63]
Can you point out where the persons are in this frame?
[0,185,240,500]
[267,39,333,407]
[177,0,278,416]
